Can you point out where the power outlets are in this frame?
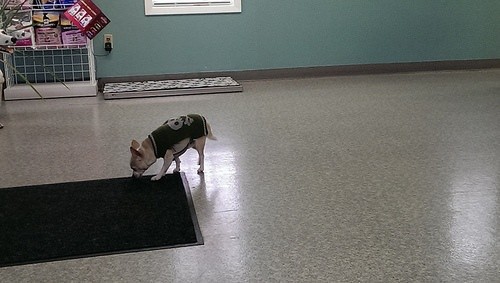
[104,34,113,49]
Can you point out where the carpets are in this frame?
[0,173,204,267]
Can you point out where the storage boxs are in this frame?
[32,11,59,29]
[60,12,80,30]
[36,28,62,50]
[6,24,34,47]
[3,10,32,27]
[62,31,87,48]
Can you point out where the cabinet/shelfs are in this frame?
[0,6,98,100]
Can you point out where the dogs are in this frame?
[130,112,218,183]
